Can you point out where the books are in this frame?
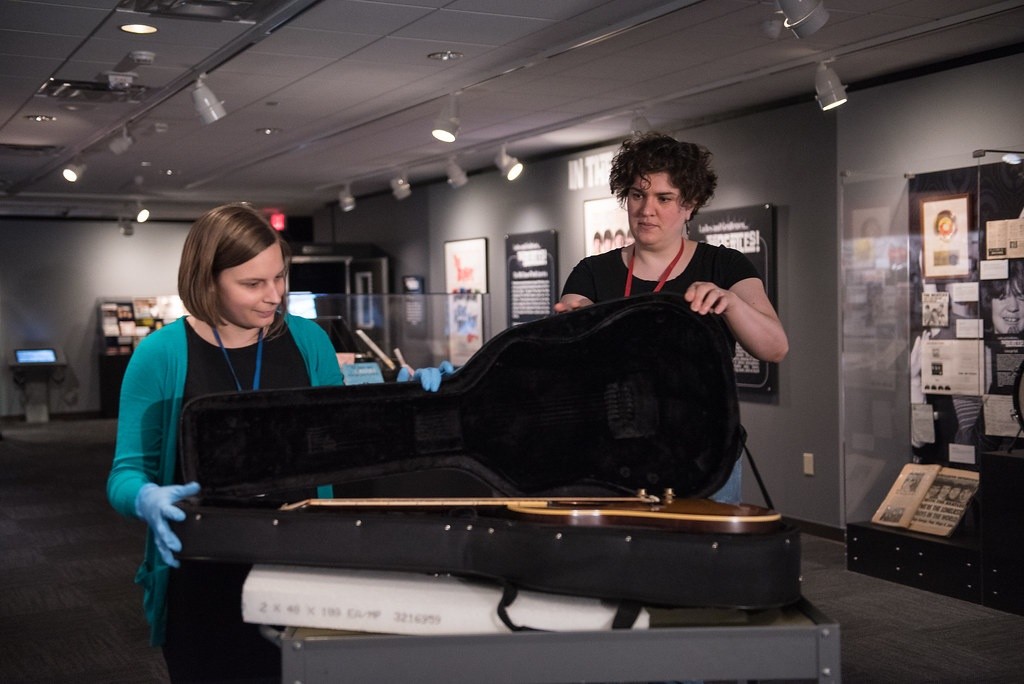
[871,463,979,537]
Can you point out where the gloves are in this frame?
[138,482,200,571]
[397,362,456,393]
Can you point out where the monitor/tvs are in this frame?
[16,348,56,363]
[288,292,317,320]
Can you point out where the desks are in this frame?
[282,597,842,684]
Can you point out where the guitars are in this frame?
[278,488,782,535]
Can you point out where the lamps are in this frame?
[391,176,412,200]
[108,126,133,155]
[339,189,356,212]
[129,202,150,223]
[118,218,133,236]
[814,57,849,112]
[62,161,87,183]
[493,143,523,181]
[191,78,226,125]
[631,115,652,142]
[446,160,469,189]
[778,0,830,41]
[432,99,463,142]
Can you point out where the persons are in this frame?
[593,229,635,255]
[107,204,454,684]
[556,129,789,506]
[911,258,1024,470]
[927,479,976,505]
[924,304,945,325]
